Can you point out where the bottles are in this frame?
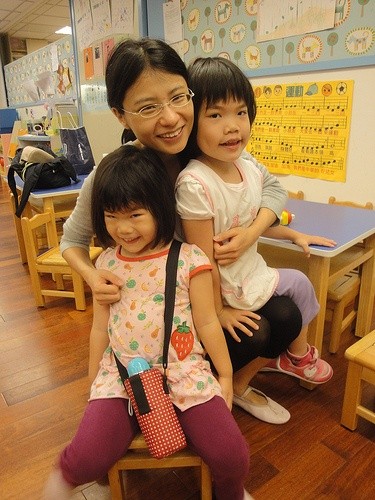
[127,357,151,377]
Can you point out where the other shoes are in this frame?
[233,384,291,424]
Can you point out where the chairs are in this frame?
[11,193,375,500]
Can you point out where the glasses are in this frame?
[117,86,195,118]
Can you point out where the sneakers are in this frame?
[276,344,333,384]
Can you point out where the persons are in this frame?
[59,36,304,426]
[173,56,342,426]
[40,145,252,500]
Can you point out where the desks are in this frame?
[260,197,375,390]
[19,135,51,149]
[4,172,91,282]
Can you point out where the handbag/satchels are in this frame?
[57,110,95,174]
[8,144,81,218]
